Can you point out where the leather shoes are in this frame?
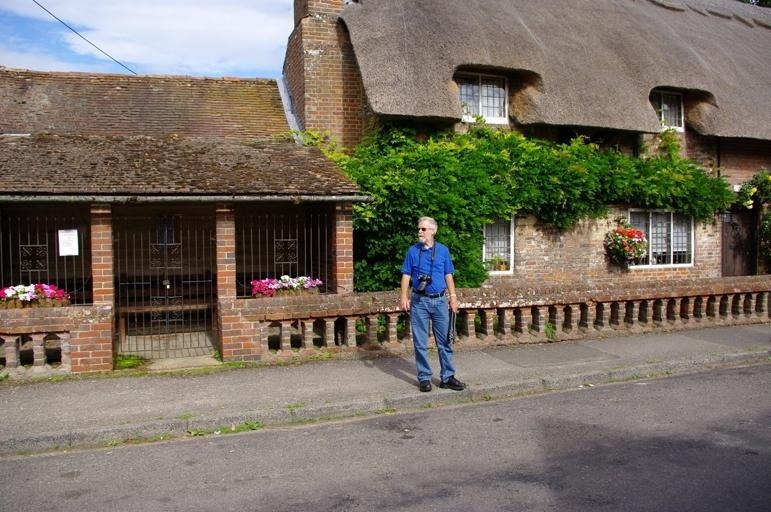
[439,375,464,390]
[420,380,432,392]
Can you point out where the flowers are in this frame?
[604,216,647,271]
[250,275,324,297]
[0,283,71,303]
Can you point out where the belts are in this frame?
[412,288,445,298]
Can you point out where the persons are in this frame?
[399,217,465,392]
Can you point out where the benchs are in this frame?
[68,283,253,344]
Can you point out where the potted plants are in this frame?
[483,256,507,270]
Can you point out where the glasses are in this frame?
[418,228,426,231]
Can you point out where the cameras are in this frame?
[417,275,432,295]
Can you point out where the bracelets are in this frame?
[451,294,457,296]
